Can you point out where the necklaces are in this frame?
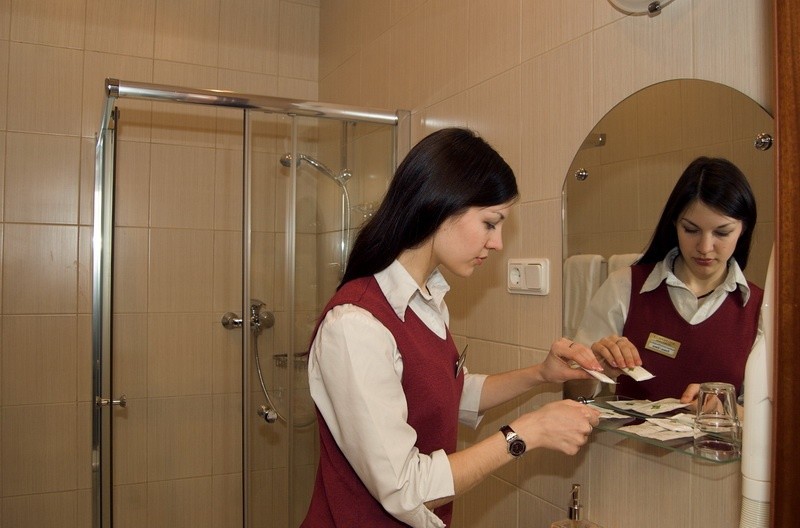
[425,284,431,297]
[671,254,721,300]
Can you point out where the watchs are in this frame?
[499,424,526,460]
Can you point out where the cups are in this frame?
[690,383,744,465]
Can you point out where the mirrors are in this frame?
[560,77,782,434]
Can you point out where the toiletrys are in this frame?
[634,396,690,417]
[593,409,633,420]
[607,396,654,413]
[648,431,697,450]
[620,419,666,439]
[672,413,715,438]
[566,362,619,389]
[548,481,599,528]
[646,417,695,435]
[622,363,657,384]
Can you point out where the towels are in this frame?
[604,253,645,280]
[563,251,609,346]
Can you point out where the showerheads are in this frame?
[278,151,340,182]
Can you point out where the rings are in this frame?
[568,342,575,348]
[615,339,624,343]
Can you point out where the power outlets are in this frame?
[502,258,527,295]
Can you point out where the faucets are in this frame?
[249,296,265,339]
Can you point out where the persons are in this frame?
[301,125,604,528]
[564,155,764,425]
